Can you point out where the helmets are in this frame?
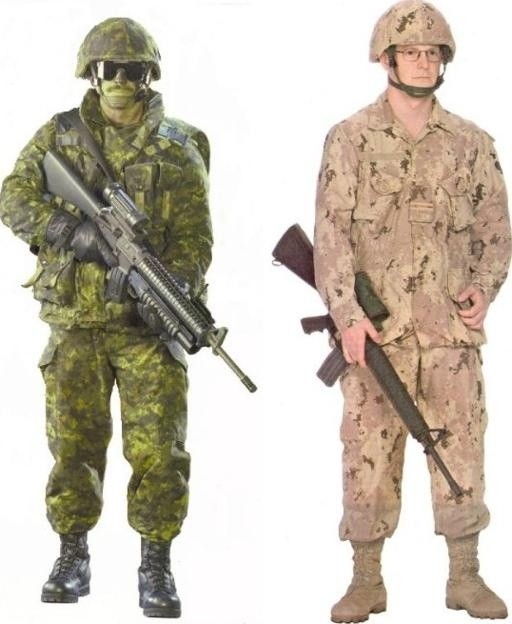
[371,0,457,62]
[77,19,162,79]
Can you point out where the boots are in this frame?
[446,532,508,618]
[138,537,182,618]
[41,532,91,602]
[330,539,386,623]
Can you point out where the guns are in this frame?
[40,150,258,392]
[271,225,467,499]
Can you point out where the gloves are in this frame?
[46,209,118,267]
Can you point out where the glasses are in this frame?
[396,47,442,64]
[94,60,143,81]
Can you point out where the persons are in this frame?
[310,2,512,624]
[2,16,214,614]
